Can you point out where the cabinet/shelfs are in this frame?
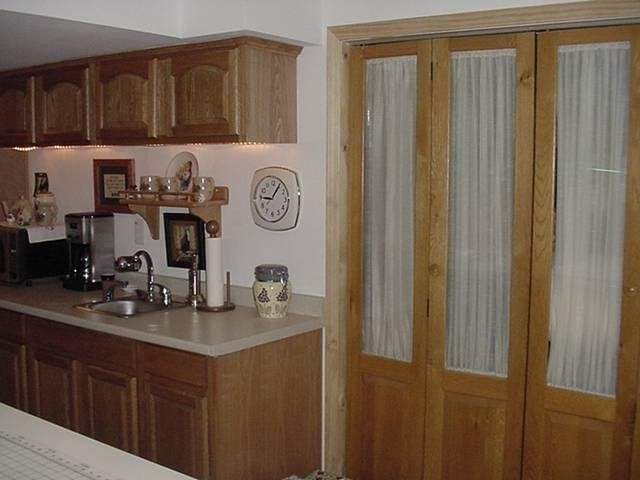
[1,58,91,148]
[0,306,80,434]
[80,326,332,479]
[91,36,301,144]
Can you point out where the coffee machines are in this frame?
[62,211,116,293]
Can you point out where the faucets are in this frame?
[132,249,156,303]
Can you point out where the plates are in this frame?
[165,151,198,194]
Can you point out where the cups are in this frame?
[192,176,215,205]
[158,177,181,198]
[100,272,117,301]
[140,175,161,199]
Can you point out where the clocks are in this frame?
[248,167,302,232]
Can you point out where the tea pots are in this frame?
[1,194,35,226]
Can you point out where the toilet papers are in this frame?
[203,237,225,309]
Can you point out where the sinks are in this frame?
[90,297,162,320]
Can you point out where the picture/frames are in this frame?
[162,212,206,270]
[92,159,137,214]
